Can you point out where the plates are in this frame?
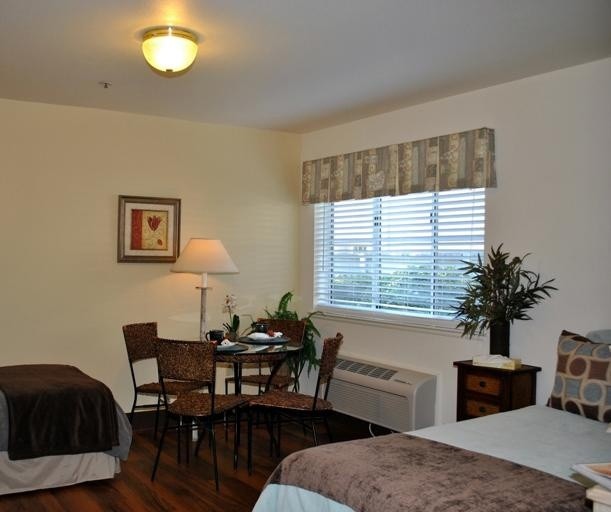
[218,345,235,348]
[247,334,277,341]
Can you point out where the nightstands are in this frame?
[453,359,542,421]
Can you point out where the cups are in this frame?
[254,322,271,335]
[206,329,225,345]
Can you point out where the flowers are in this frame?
[220,293,240,333]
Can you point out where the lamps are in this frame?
[140,28,196,72]
[170,237,239,340]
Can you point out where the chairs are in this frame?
[248,332,343,474]
[149,336,249,492]
[224,318,307,441]
[122,322,204,436]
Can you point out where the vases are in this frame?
[225,332,237,342]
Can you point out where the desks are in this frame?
[197,335,303,474]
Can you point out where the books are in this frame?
[570,461,611,511]
[238,344,270,354]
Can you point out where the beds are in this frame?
[254,404,610,511]
[1,364,130,498]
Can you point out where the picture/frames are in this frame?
[117,195,180,262]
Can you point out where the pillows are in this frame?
[546,329,611,423]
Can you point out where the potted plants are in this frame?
[448,241,557,357]
[241,292,324,394]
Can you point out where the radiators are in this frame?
[320,355,437,432]
